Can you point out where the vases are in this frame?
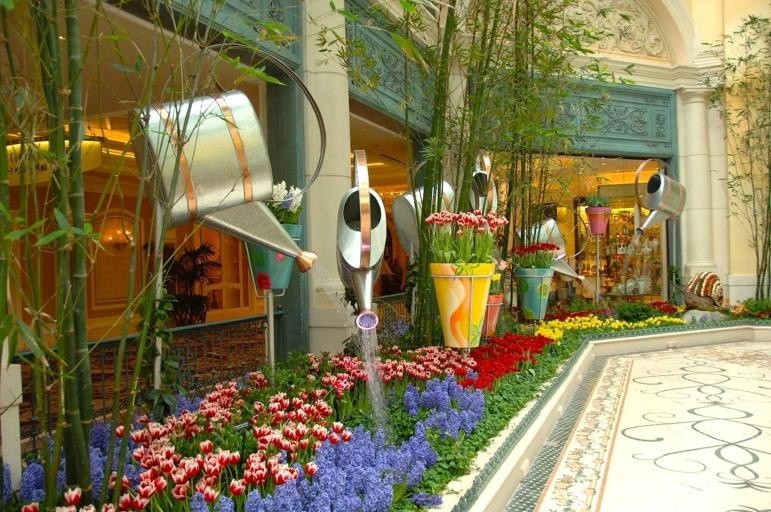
[245,221,305,290]
[430,261,498,349]
[514,268,557,321]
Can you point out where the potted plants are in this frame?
[585,193,612,233]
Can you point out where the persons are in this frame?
[381,256,403,295]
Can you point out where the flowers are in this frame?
[422,207,510,265]
[265,180,305,225]
[512,241,561,269]
